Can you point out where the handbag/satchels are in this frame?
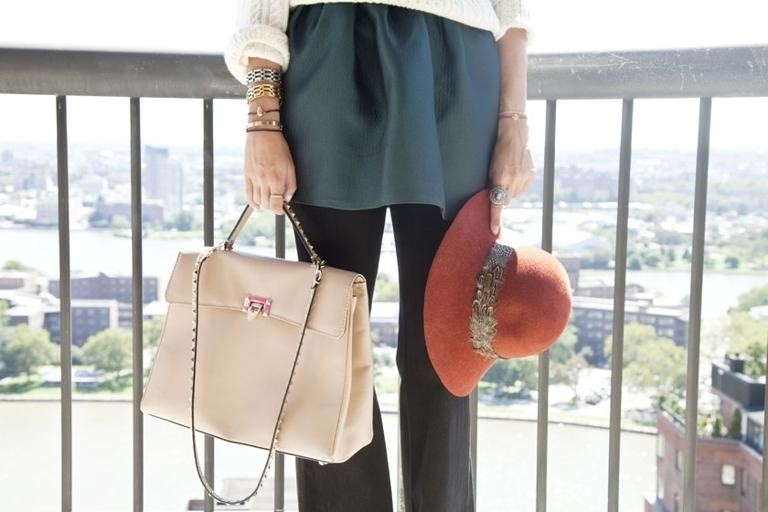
[140,202,374,465]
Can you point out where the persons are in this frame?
[219,0,537,510]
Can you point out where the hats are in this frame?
[424,190,572,397]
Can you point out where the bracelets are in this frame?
[244,128,284,134]
[498,113,528,121]
[244,119,283,129]
[248,103,280,119]
[241,68,284,85]
[245,84,284,106]
[499,110,528,115]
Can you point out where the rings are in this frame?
[270,193,285,199]
[487,185,508,206]
